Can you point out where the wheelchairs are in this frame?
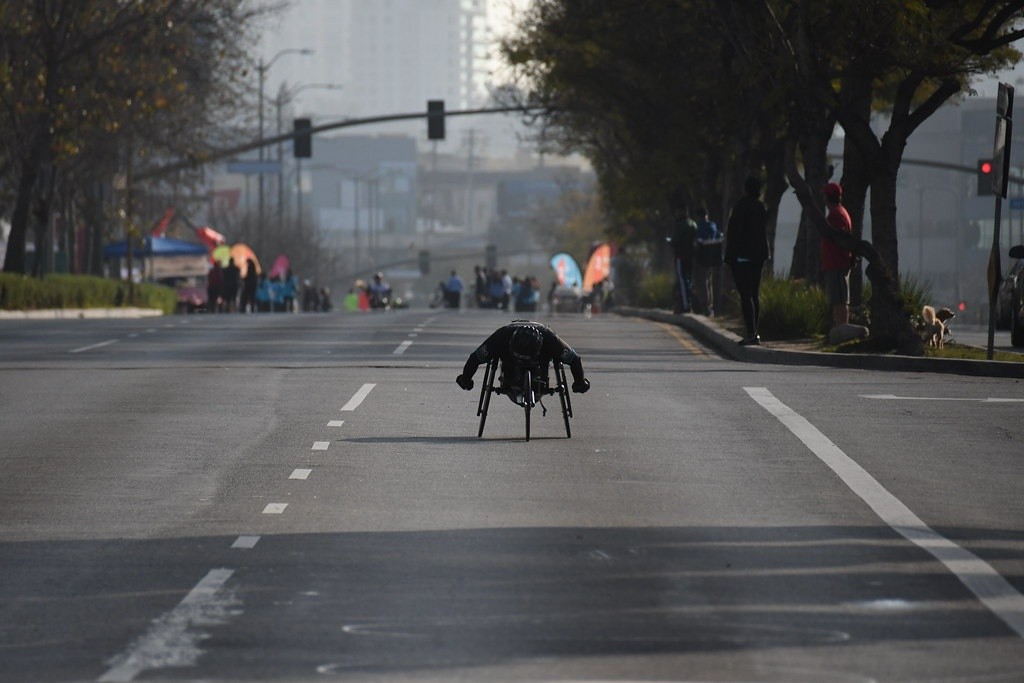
[475,346,574,442]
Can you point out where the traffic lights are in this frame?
[977,158,996,197]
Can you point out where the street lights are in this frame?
[256,47,358,269]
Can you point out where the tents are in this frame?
[104,235,209,291]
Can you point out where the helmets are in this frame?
[510,326,543,360]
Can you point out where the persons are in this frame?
[691,207,722,316]
[203,258,333,313]
[723,176,771,346]
[820,183,859,344]
[430,266,615,313]
[456,319,590,395]
[344,271,392,312]
[667,205,697,315]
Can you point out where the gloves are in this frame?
[574,380,588,393]
[456,373,473,390]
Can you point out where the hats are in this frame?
[821,183,842,195]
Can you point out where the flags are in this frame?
[583,244,612,296]
[550,253,583,297]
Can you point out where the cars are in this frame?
[995,244,1024,347]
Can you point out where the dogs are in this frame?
[924,304,955,348]
